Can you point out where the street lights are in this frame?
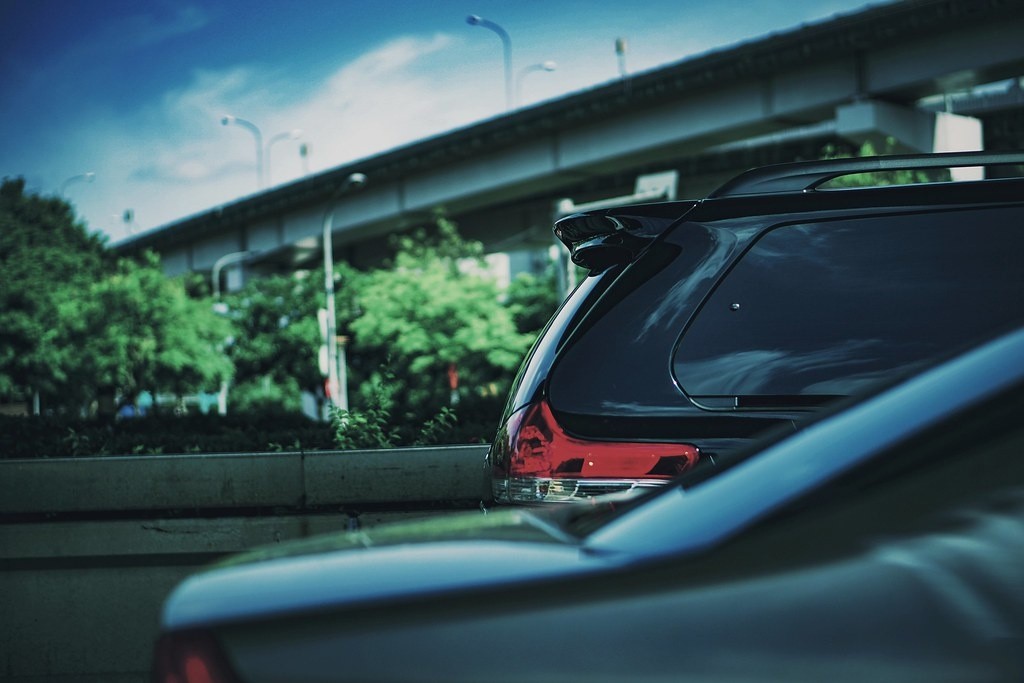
[218,113,312,190]
[467,11,555,112]
[324,171,366,426]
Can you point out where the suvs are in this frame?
[481,151,1024,510]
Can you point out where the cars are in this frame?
[148,330,1024,683]
